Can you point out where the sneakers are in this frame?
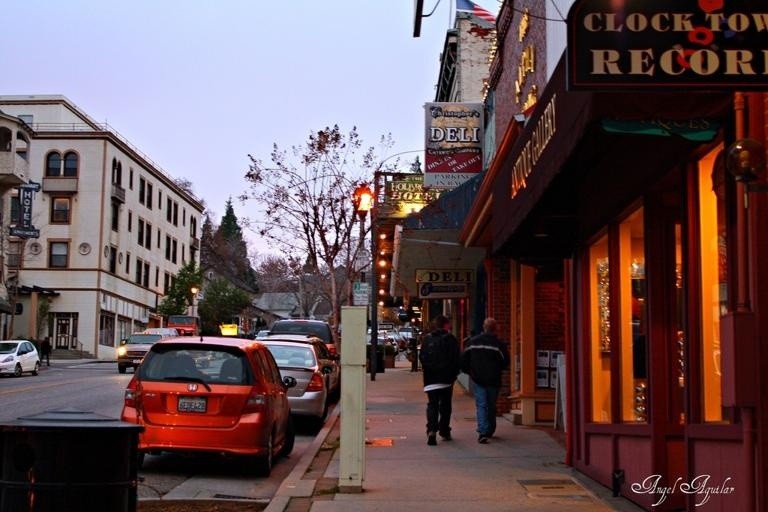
[478,433,488,443]
[427,431,451,445]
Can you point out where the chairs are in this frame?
[168,354,242,381]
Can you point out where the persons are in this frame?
[461,317,509,441]
[418,315,462,446]
[39,336,53,366]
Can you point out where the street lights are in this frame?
[352,183,373,282]
[191,285,198,316]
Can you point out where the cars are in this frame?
[256,318,341,422]
[122,335,295,478]
[118,332,170,372]
[0,340,41,377]
[368,327,420,355]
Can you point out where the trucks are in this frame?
[167,316,200,336]
